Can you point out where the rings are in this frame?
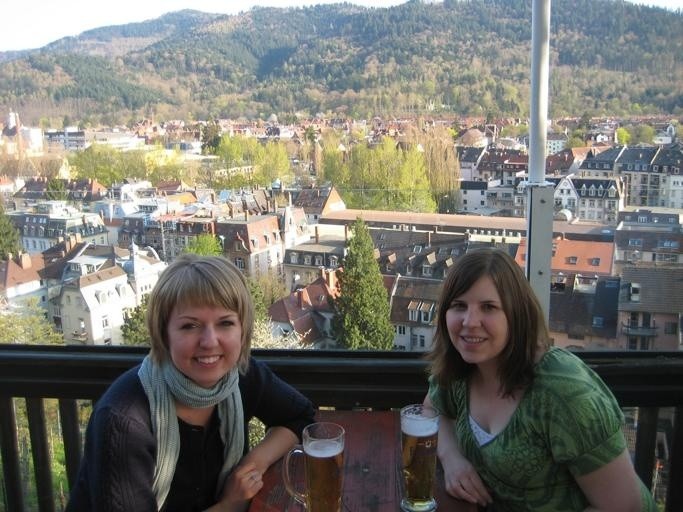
[252,475,257,482]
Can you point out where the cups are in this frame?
[282,422,346,512]
[400,403,440,512]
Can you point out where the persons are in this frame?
[63,254,316,512]
[421,247,657,512]
